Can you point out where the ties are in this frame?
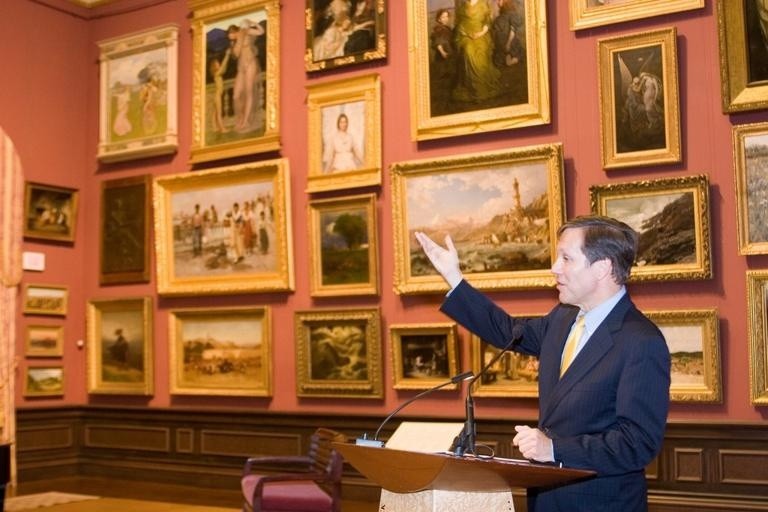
[559,316,585,380]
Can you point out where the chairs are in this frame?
[240,427,347,512]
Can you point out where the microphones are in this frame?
[450,325,525,457]
[356,371,474,448]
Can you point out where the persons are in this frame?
[512,350,540,382]
[209,48,232,134]
[112,86,133,136]
[640,72,662,129]
[620,76,649,148]
[312,1,376,63]
[414,214,671,511]
[182,355,261,375]
[138,70,159,135]
[107,329,129,371]
[179,193,275,270]
[430,0,527,118]
[324,113,366,174]
[228,19,266,134]
